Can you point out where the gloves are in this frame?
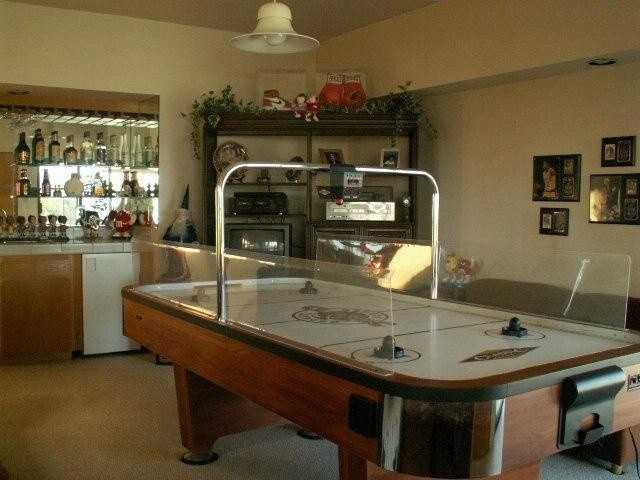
[319,73,343,108]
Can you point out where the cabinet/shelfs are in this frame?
[0,79,165,241]
[201,108,423,268]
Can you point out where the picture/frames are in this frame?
[530,132,640,236]
[378,147,403,170]
[318,147,346,166]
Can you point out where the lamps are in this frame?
[229,0,323,61]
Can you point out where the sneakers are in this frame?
[262,90,292,110]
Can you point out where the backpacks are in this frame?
[341,73,367,107]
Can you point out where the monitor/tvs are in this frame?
[223,222,292,279]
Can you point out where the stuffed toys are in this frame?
[162,184,198,244]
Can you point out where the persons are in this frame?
[0,215,68,242]
[383,155,395,168]
[329,152,341,164]
[292,93,308,118]
[541,161,558,198]
[304,95,319,121]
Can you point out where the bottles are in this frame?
[14,127,158,198]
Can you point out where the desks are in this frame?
[117,275,640,478]
